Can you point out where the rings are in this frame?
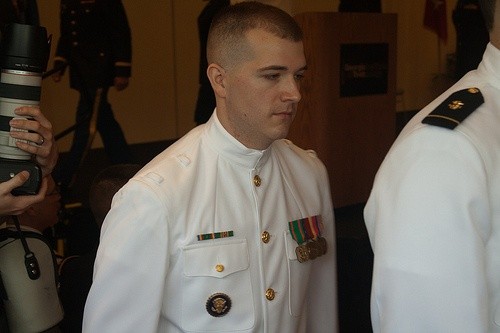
[35,135,45,144]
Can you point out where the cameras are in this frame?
[0,24,52,196]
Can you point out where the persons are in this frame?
[0,0,40,25]
[82,1,341,333]
[0,102,142,333]
[451,0,490,80]
[194,0,229,126]
[364,0,500,333]
[50,0,147,197]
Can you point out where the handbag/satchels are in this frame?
[0,215,64,333]
